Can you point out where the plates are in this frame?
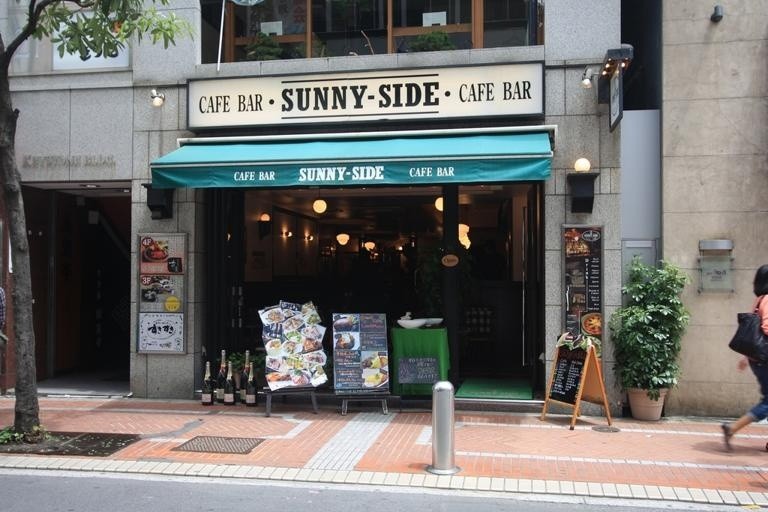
[396,317,445,328]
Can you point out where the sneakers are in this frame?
[721,424,733,450]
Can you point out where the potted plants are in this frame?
[607,253,693,421]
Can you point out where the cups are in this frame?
[464,308,493,336]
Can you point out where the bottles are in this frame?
[224,361,236,407]
[201,361,213,406]
[216,349,228,404]
[239,350,251,403]
[244,362,257,406]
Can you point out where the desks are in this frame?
[391,326,449,396]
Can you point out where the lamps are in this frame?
[580,63,601,89]
[257,213,272,240]
[312,188,327,214]
[149,88,165,106]
[281,231,315,241]
[565,157,602,214]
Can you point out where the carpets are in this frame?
[455,374,533,399]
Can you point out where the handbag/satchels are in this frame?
[728,313,767,361]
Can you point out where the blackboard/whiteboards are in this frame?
[581,345,614,405]
[546,335,592,410]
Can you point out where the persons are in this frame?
[719,264,768,455]
